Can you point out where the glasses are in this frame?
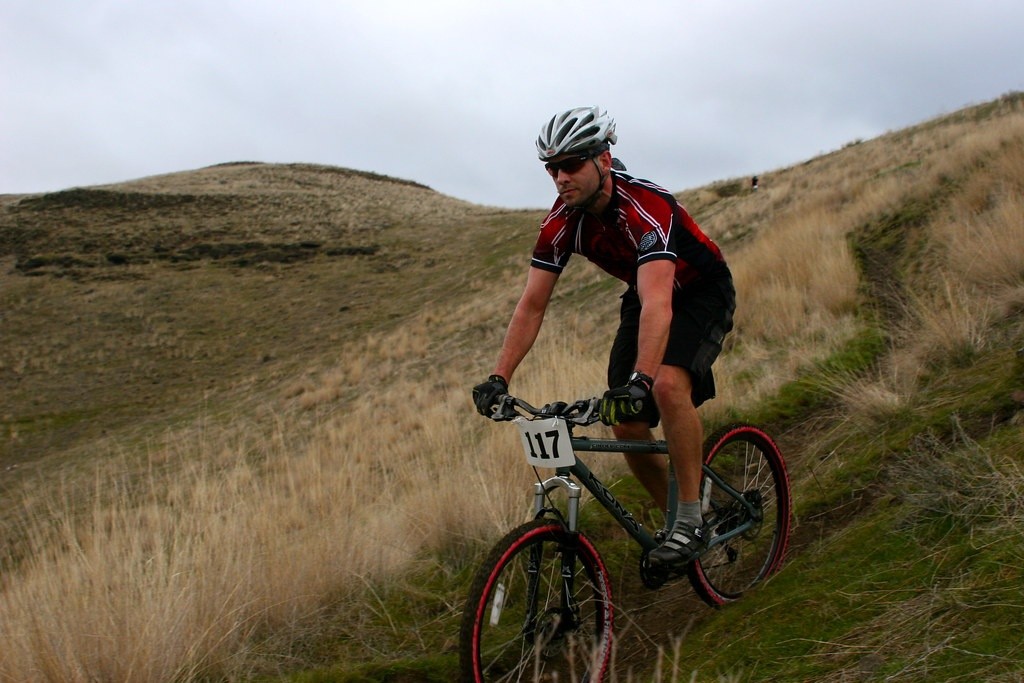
[544,146,606,178]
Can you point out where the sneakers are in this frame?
[653,528,670,545]
[650,520,712,569]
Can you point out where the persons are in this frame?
[474,111,738,569]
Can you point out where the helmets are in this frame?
[534,104,617,162]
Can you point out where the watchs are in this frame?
[628,369,656,388]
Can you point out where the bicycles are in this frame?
[457,394,793,683]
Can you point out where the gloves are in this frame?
[598,380,653,427]
[473,374,508,420]
